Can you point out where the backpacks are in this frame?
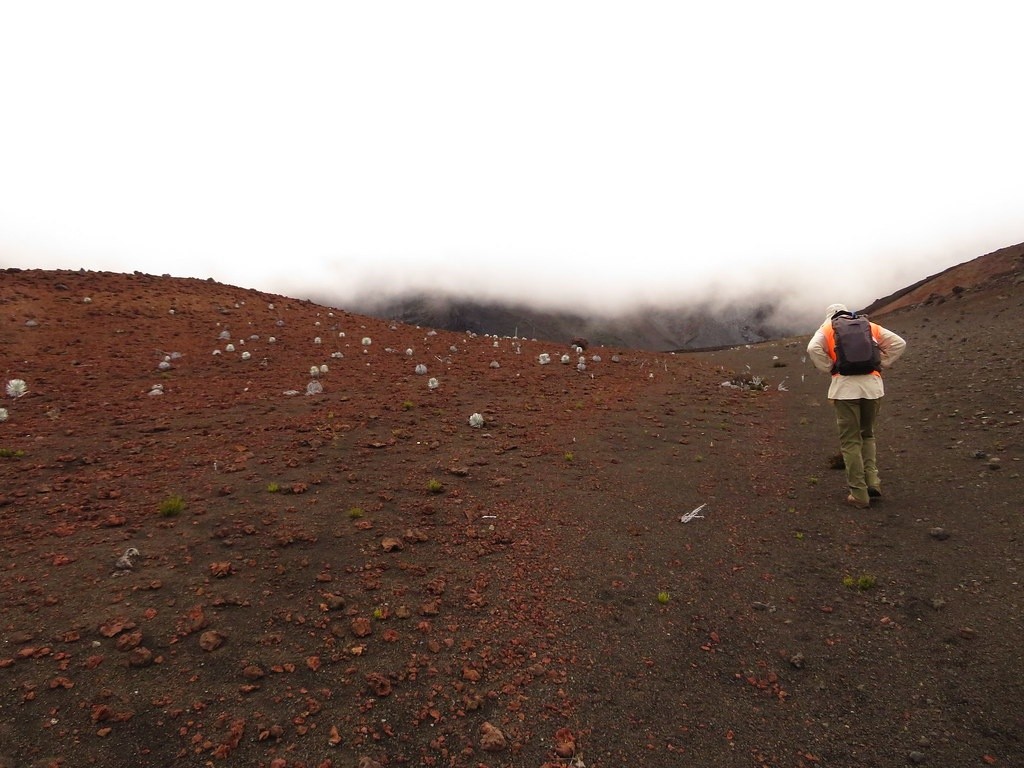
[830,314,882,376]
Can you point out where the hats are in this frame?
[820,304,850,326]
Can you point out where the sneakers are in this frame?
[847,494,871,510]
[866,485,882,496]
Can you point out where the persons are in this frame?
[807,304,907,508]
